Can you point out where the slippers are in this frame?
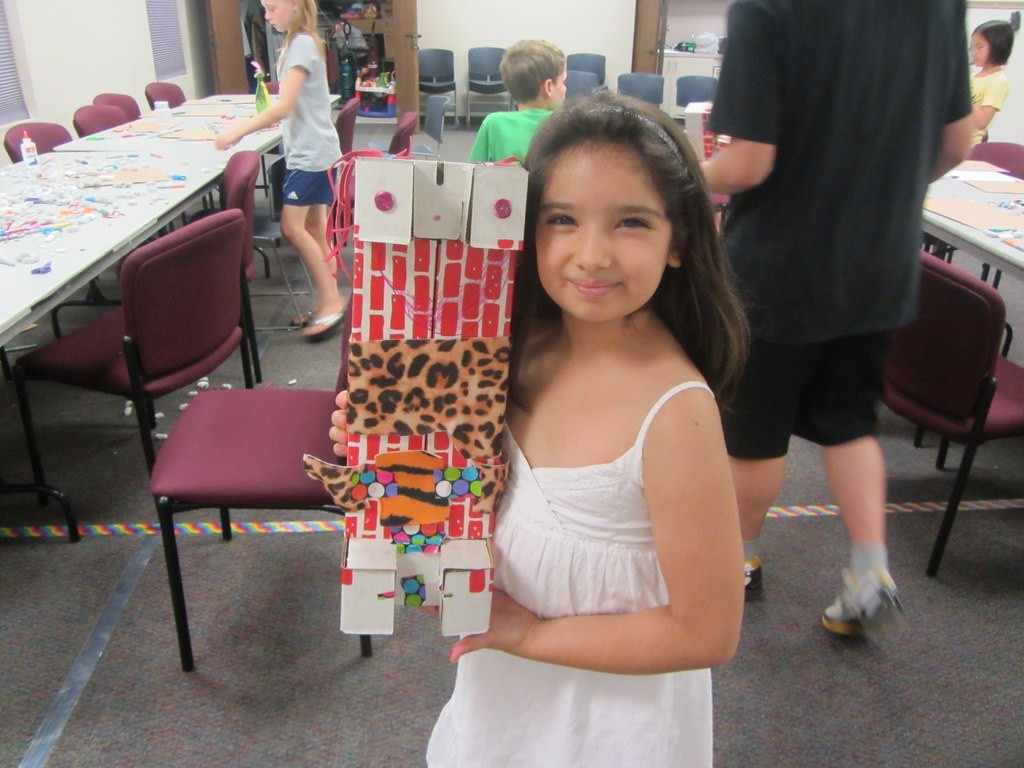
[289,311,315,327]
[299,305,346,341]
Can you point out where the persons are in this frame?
[213,0,348,342]
[467,39,568,168]
[697,0,973,633]
[328,90,745,768]
[969,19,1014,148]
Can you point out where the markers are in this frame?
[87,201,109,214]
[86,198,112,204]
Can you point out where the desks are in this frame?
[920,170,1024,358]
[52,94,342,279]
[0,149,235,540]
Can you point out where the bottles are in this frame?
[20,131,38,165]
[255,73,270,113]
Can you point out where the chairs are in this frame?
[467,47,511,129]
[563,54,718,133]
[148,290,374,671]
[879,140,1024,576]
[419,49,461,131]
[12,208,254,506]
[0,82,451,384]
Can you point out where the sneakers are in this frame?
[822,564,905,637]
[743,555,763,601]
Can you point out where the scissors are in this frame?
[32,261,51,274]
